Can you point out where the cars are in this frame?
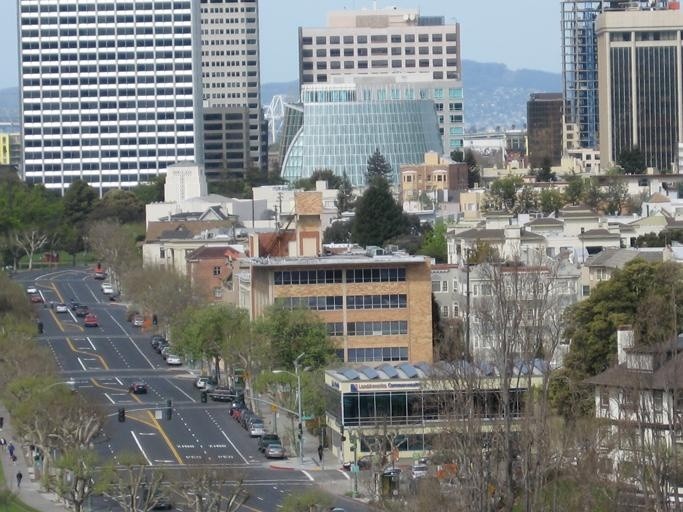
[197,377,285,459]
[56,301,98,327]
[344,455,429,481]
[125,309,144,326]
[26,286,41,303]
[94,273,114,295]
[151,336,182,365]
[131,382,148,394]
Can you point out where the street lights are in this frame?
[271,371,303,465]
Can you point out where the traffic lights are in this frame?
[298,424,302,439]
[119,409,125,423]
[341,436,346,441]
[153,315,156,324]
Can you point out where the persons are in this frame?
[0,437,22,487]
